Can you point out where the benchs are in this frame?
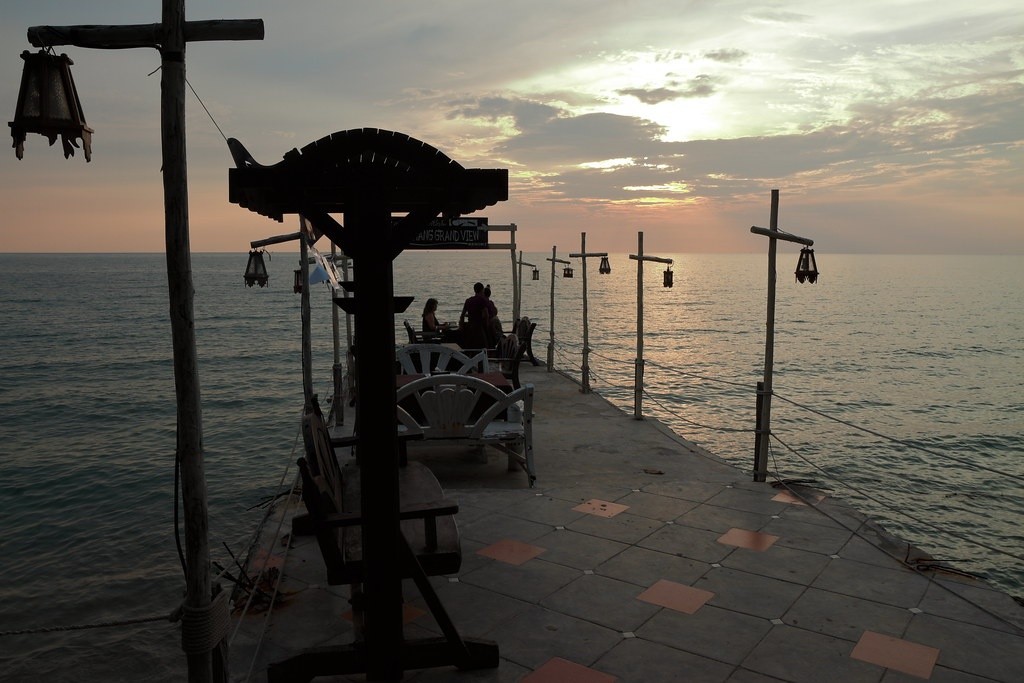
[512,316,537,366]
[397,374,538,489]
[460,335,525,390]
[396,344,489,373]
[297,394,464,643]
[403,319,432,344]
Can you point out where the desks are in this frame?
[397,374,514,399]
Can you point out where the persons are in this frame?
[458,282,498,331]
[422,299,449,332]
